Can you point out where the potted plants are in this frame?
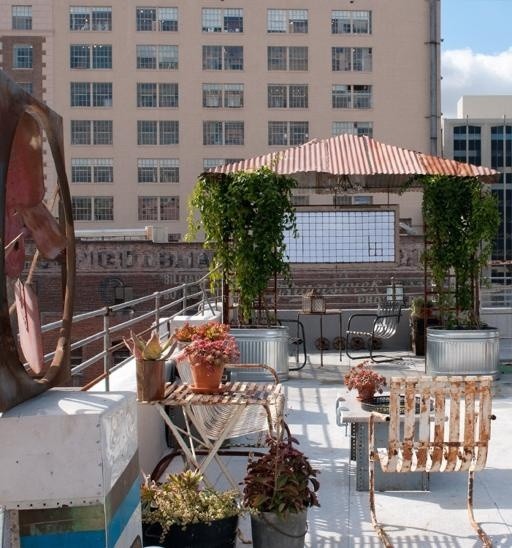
[183,153,298,382]
[398,173,500,381]
[239,436,321,548]
[120,325,176,401]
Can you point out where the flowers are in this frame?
[174,321,230,343]
[138,468,262,543]
[185,333,239,376]
[343,360,386,390]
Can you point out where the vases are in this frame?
[190,364,225,387]
[358,385,373,400]
[144,515,238,547]
[177,341,192,381]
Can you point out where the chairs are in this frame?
[165,353,291,462]
[345,299,402,363]
[253,316,306,370]
[367,375,497,548]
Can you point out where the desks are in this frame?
[337,393,448,491]
[136,382,287,494]
[296,309,342,368]
